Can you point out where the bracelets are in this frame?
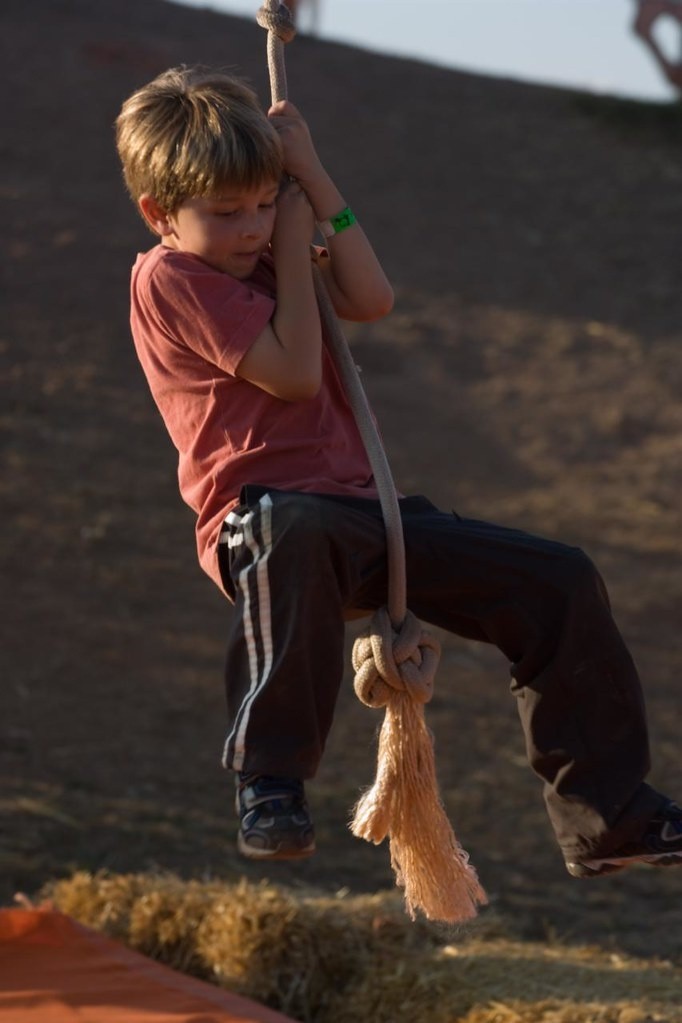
[321,207,357,238]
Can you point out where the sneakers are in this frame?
[235,773,316,861]
[561,797,682,880]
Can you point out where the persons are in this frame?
[116,63,682,873]
